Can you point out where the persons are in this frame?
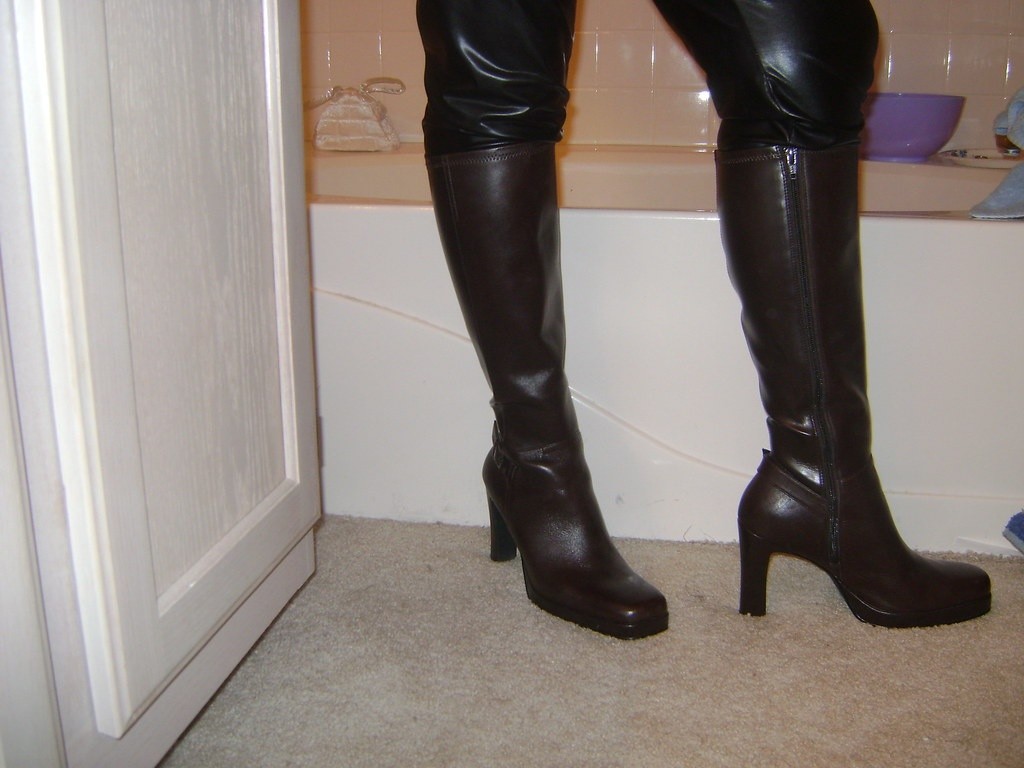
[413,0,992,639]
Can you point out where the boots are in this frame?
[714,131,994,628]
[424,140,669,642]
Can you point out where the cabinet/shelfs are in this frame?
[0,0,324,768]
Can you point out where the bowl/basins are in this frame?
[855,91,967,164]
